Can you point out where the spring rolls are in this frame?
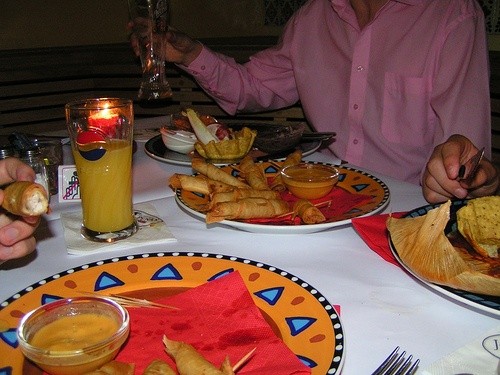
[1,181,50,217]
[170,150,325,224]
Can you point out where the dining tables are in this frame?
[0,98,500,375]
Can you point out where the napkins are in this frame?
[61,210,177,256]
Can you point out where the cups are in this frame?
[127,0,175,100]
[32,138,62,196]
[65,97,139,242]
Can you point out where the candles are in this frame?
[88,113,123,136]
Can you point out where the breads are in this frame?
[457,195,500,258]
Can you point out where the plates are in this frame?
[0,252,344,375]
[144,134,322,166]
[174,159,390,234]
[388,198,500,316]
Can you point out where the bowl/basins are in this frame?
[252,122,307,153]
[160,129,199,154]
[280,162,339,199]
[17,296,129,375]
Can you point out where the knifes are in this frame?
[457,147,485,182]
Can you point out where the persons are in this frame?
[0,157,41,266]
[126,0,491,185]
[421,134,500,204]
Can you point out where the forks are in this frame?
[371,346,420,375]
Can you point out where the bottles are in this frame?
[58,145,82,203]
[0,148,50,202]
